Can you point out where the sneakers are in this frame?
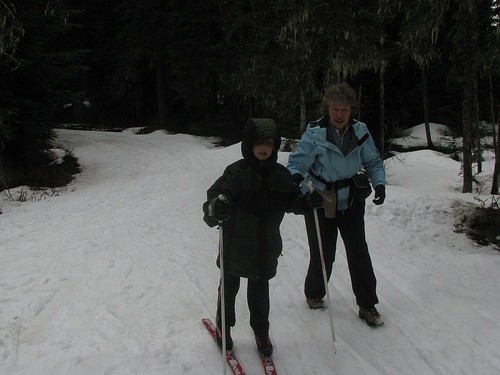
[358,305,384,327]
[255,329,273,356]
[215,324,235,351]
[306,297,325,309]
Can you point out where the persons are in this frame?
[284,83,386,328]
[204,118,323,356]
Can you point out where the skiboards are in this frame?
[202,319,277,375]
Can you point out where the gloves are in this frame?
[302,189,323,208]
[208,195,227,217]
[373,185,386,205]
[292,173,305,185]
